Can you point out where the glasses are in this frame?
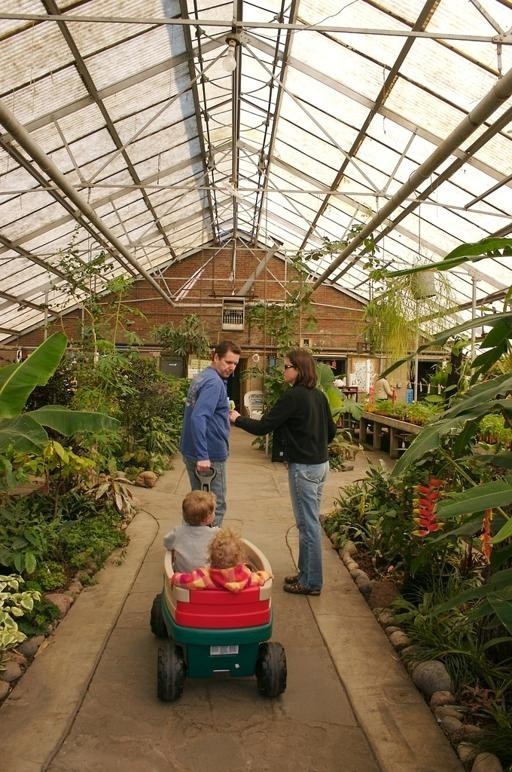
[285,365,295,369]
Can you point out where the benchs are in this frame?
[244,391,263,420]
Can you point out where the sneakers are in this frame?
[283,575,321,595]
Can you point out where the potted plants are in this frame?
[354,246,466,388]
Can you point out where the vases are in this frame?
[404,556,458,612]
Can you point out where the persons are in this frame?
[375,376,393,402]
[181,340,241,526]
[230,349,337,596]
[164,490,220,573]
[172,527,274,593]
